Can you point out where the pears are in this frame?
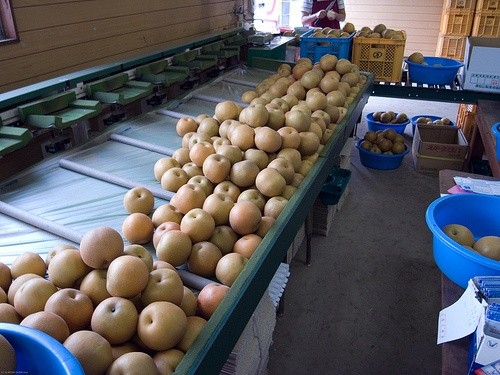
[409,53,444,66]
[310,23,354,46]
[444,223,500,261]
[327,10,336,20]
[359,129,406,155]
[281,31,302,47]
[0,55,371,375]
[354,24,404,40]
[413,116,451,126]
[372,111,407,124]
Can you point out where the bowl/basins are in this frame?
[411,116,456,137]
[490,122,500,162]
[355,138,411,170]
[0,322,84,375]
[365,112,410,135]
[405,57,465,85]
[425,193,500,290]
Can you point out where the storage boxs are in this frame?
[299,27,407,83]
[280,136,356,266]
[435,0,500,94]
[411,102,474,171]
[437,276,500,375]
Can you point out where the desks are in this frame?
[467,99,500,178]
[439,169,500,375]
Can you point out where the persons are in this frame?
[301,0,346,29]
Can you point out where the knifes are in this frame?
[314,1,336,25]
